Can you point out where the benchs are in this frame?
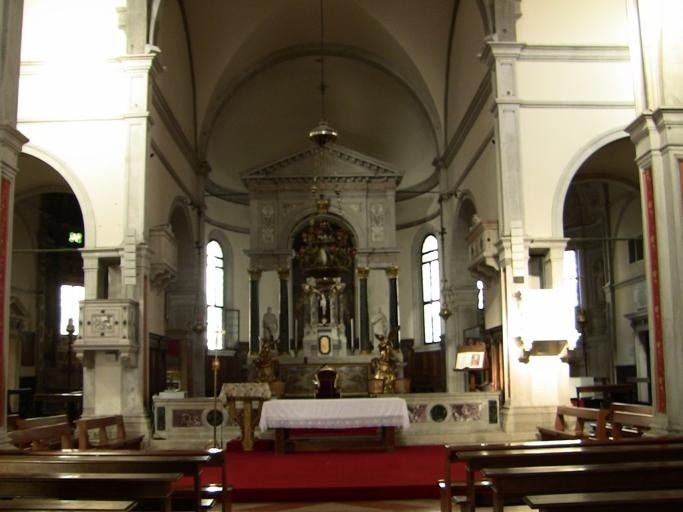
[434,400,683,512]
[0,414,237,511]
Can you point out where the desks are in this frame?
[259,396,409,454]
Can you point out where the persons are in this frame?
[370,306,388,339]
[262,306,276,349]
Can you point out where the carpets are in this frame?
[170,444,480,501]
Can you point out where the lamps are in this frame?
[307,0,338,146]
